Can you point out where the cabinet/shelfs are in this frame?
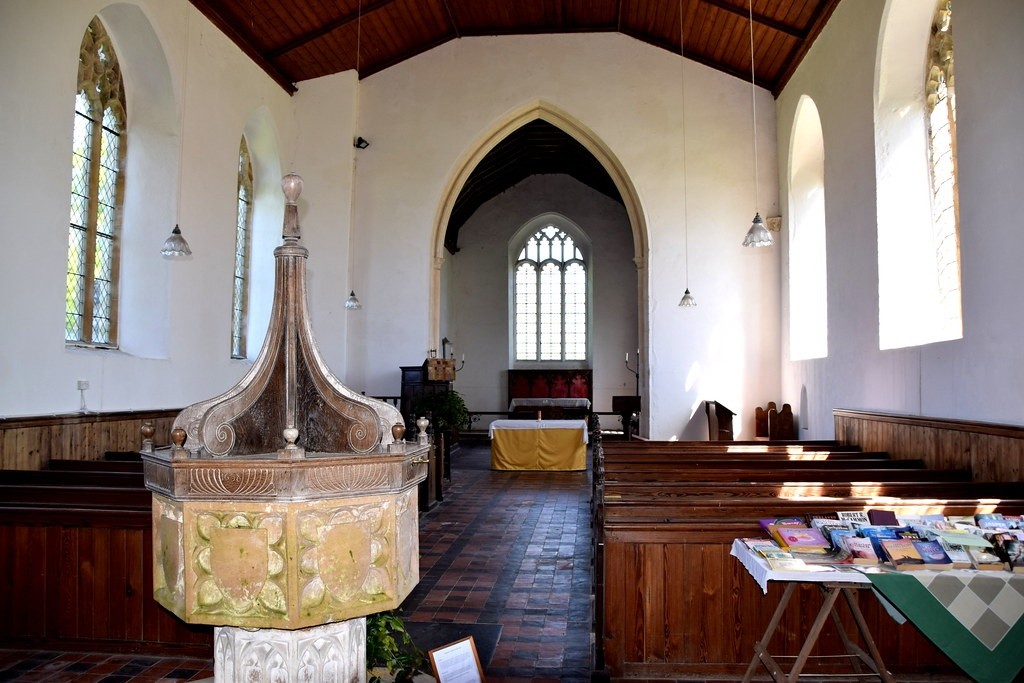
[508,398,590,419]
[399,366,451,460]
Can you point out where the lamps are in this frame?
[743,0,774,249]
[345,0,361,311]
[160,0,191,258]
[678,0,697,307]
[354,136,369,149]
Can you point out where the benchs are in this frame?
[591,407,1024,683]
[0,391,453,644]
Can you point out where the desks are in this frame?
[730,537,1024,683]
[488,419,589,472]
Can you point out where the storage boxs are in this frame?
[427,356,456,381]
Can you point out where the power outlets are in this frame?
[78,381,89,390]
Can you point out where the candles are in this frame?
[538,411,542,420]
[626,352,628,360]
[462,354,464,361]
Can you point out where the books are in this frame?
[743,510,1024,573]
